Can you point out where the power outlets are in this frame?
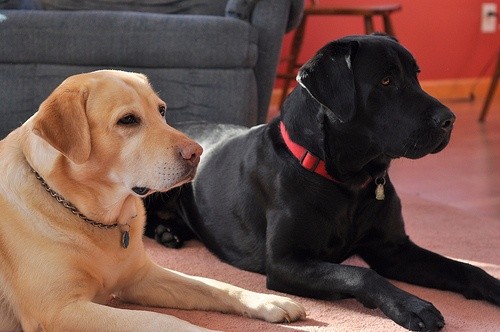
[480,3,497,32]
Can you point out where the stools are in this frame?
[275,5,402,112]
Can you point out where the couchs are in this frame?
[0,0,305,141]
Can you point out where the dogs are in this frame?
[1,68,307,332]
[142,30,500,332]
